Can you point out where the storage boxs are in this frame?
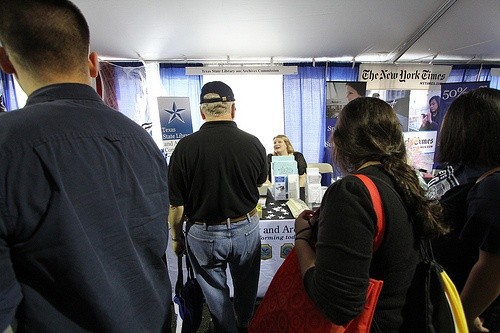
[256,204,262,218]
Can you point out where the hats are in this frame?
[200,81,235,104]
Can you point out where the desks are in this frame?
[166,201,296,297]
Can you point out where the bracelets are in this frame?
[172,234,184,241]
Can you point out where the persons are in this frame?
[295,97,450,333]
[168,81,269,333]
[440,85,500,333]
[0,0,177,333]
[267,135,307,202]
[419,96,440,130]
[346,82,366,101]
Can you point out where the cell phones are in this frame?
[421,114,426,118]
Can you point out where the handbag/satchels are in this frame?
[173,252,203,333]
[426,260,470,333]
[246,174,384,333]
